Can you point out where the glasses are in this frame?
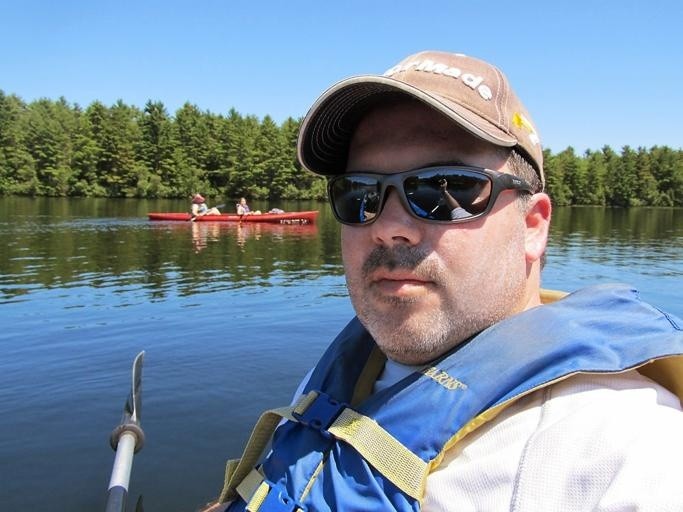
[326,165,534,228]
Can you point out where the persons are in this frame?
[222,47,681,512]
[236,194,259,217]
[191,194,220,216]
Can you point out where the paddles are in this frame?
[104,350,144,512]
[191,204,226,220]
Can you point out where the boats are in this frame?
[148,211,321,223]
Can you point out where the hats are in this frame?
[295,51,545,193]
[192,195,205,203]
